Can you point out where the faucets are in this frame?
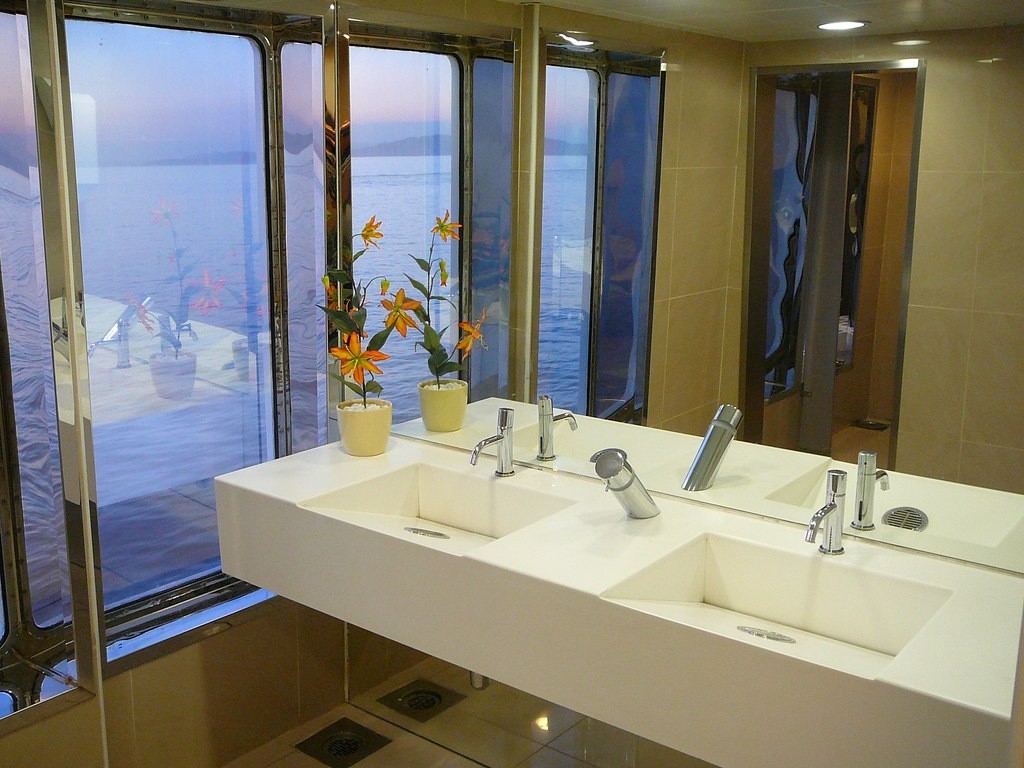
[804,468,848,554]
[849,449,890,528]
[470,406,515,475]
[535,394,579,462]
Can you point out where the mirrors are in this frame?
[334,1,1023,577]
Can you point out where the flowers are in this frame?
[380,210,487,387]
[120,194,268,360]
[312,214,422,410]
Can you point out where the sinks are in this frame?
[295,462,579,551]
[765,457,1024,547]
[493,411,721,479]
[599,530,954,683]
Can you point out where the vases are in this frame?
[233,340,267,383]
[335,400,392,456]
[415,380,468,433]
[150,352,196,397]
